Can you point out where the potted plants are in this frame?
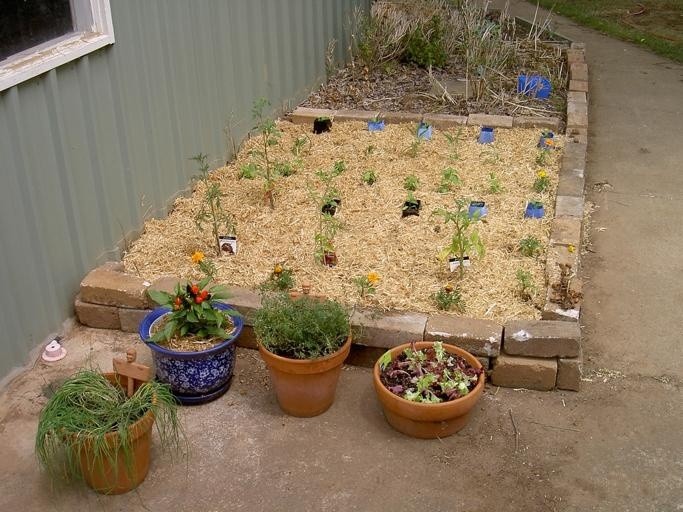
[30,371,190,511]
[245,285,487,439]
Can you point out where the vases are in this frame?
[139,300,244,406]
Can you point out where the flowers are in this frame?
[144,273,248,347]
